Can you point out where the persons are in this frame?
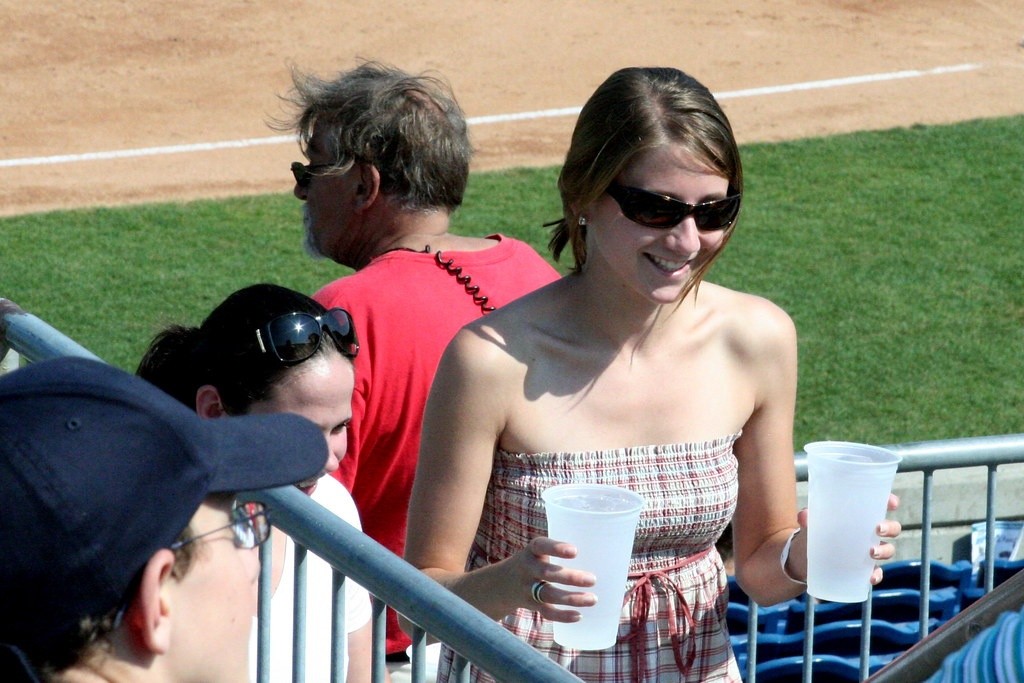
[134,282,392,683]
[264,52,565,663]
[0,356,329,683]
[393,66,901,683]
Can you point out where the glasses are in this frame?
[234,308,360,362]
[605,179,742,230]
[291,162,357,187]
[114,501,273,630]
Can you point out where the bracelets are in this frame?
[778,526,810,585]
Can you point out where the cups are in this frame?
[541,483,646,650]
[803,441,903,604]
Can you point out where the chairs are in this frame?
[727,559,1024,683]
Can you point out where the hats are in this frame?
[0,358,329,683]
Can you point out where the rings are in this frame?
[531,581,551,605]
